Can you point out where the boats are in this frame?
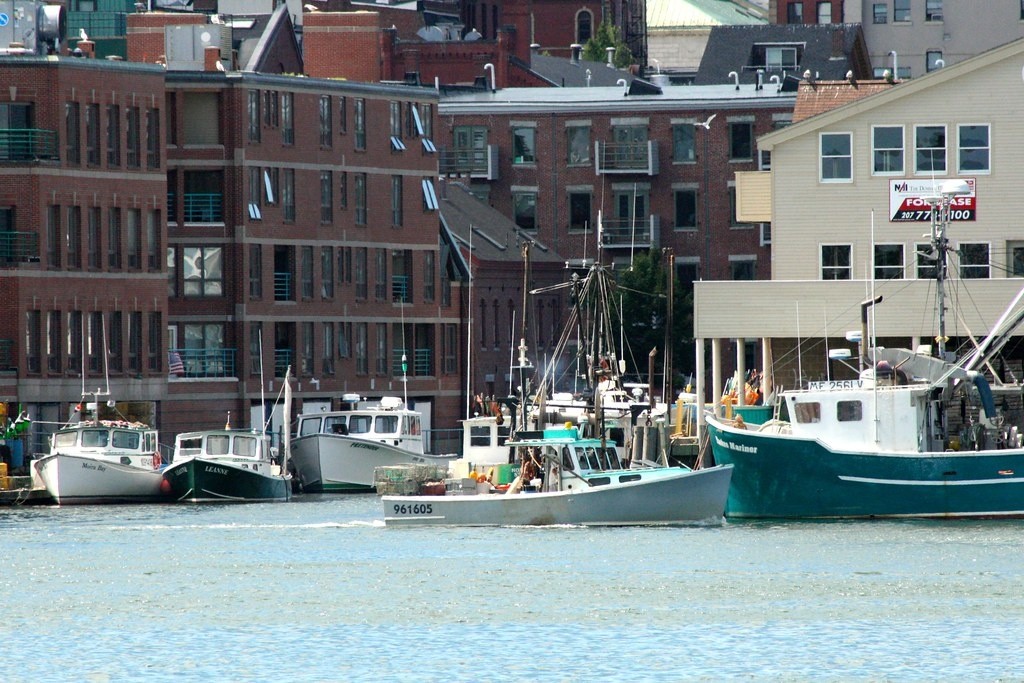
[30,143,1024,530]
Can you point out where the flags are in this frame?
[169,352,185,374]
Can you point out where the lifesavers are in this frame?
[153,452,162,469]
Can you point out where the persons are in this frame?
[733,413,748,429]
[525,457,535,482]
